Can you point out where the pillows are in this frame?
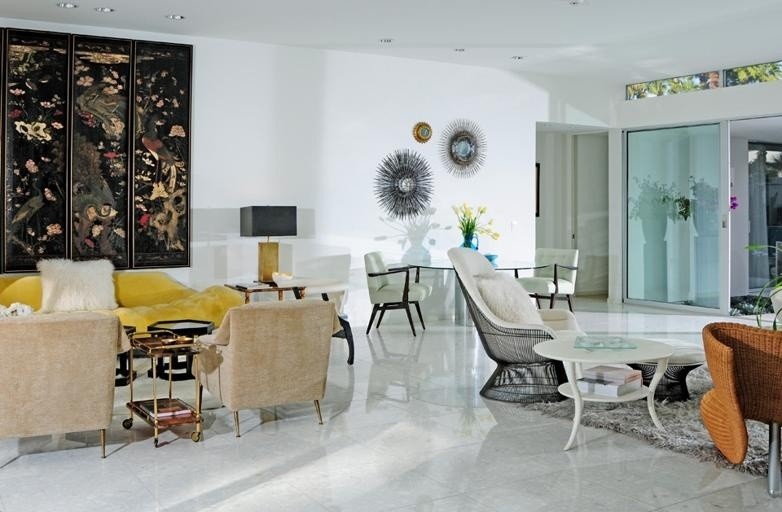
[36,255,119,315]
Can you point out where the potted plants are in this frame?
[627,174,691,244]
[689,175,739,238]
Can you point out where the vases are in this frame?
[457,238,481,252]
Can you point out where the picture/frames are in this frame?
[71,32,133,272]
[132,37,194,269]
[535,163,541,217]
[0,27,71,277]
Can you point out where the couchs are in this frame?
[473,268,549,327]
[0,267,242,338]
[191,297,335,439]
[513,246,581,317]
[362,251,434,337]
[291,253,352,317]
[1,311,121,460]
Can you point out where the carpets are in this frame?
[543,387,773,479]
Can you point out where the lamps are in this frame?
[239,205,297,284]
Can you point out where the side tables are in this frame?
[223,279,305,304]
[123,329,205,451]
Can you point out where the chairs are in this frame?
[694,320,782,497]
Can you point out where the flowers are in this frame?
[450,201,501,241]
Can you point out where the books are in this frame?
[139,398,191,418]
[576,366,643,399]
